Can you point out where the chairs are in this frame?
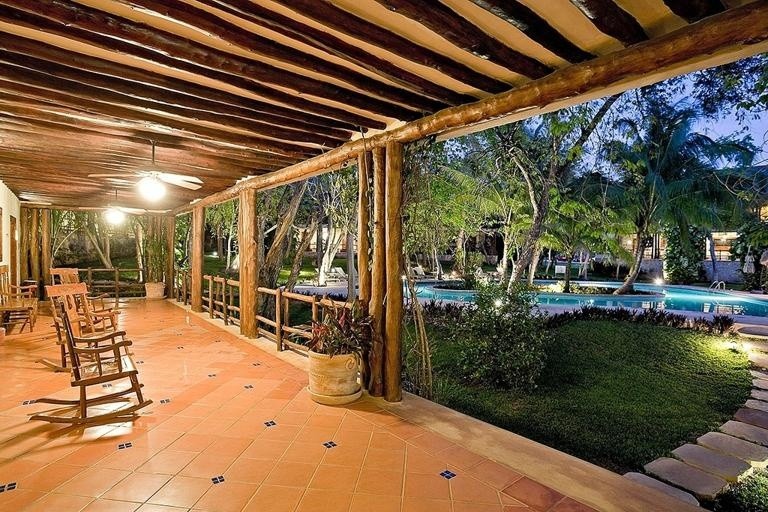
[406,267,423,281]
[415,268,436,280]
[0,259,152,427]
[314,266,348,282]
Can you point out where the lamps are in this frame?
[104,207,125,226]
[136,176,166,202]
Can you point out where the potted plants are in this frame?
[298,292,372,404]
[143,237,170,301]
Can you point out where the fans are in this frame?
[74,191,173,215]
[85,138,202,192]
